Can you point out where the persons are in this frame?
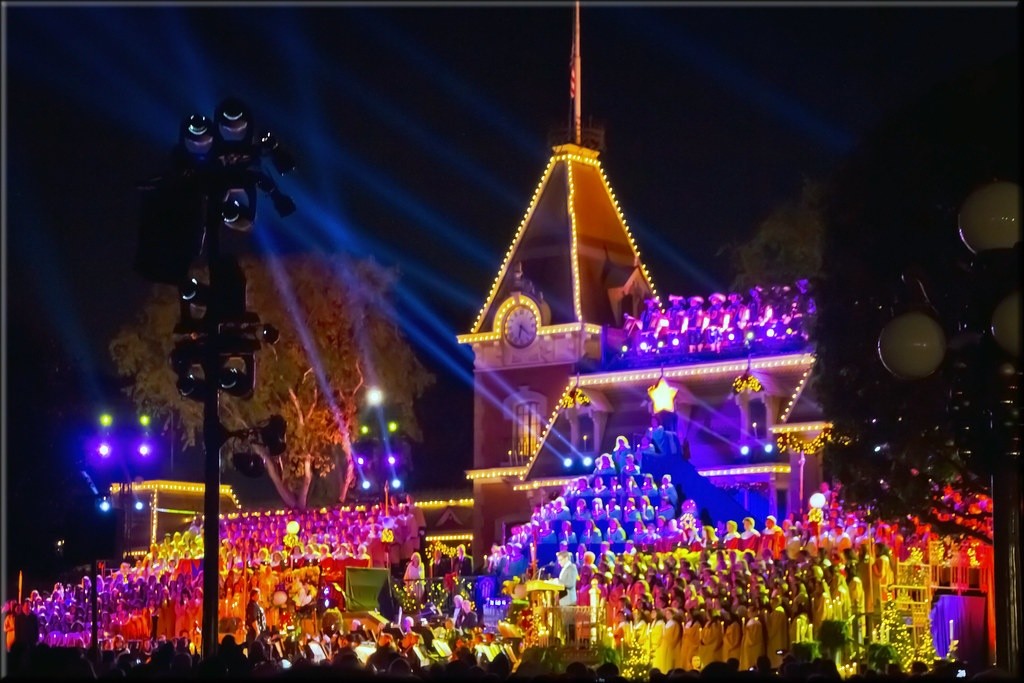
[0,417,973,683]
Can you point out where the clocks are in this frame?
[501,304,538,349]
